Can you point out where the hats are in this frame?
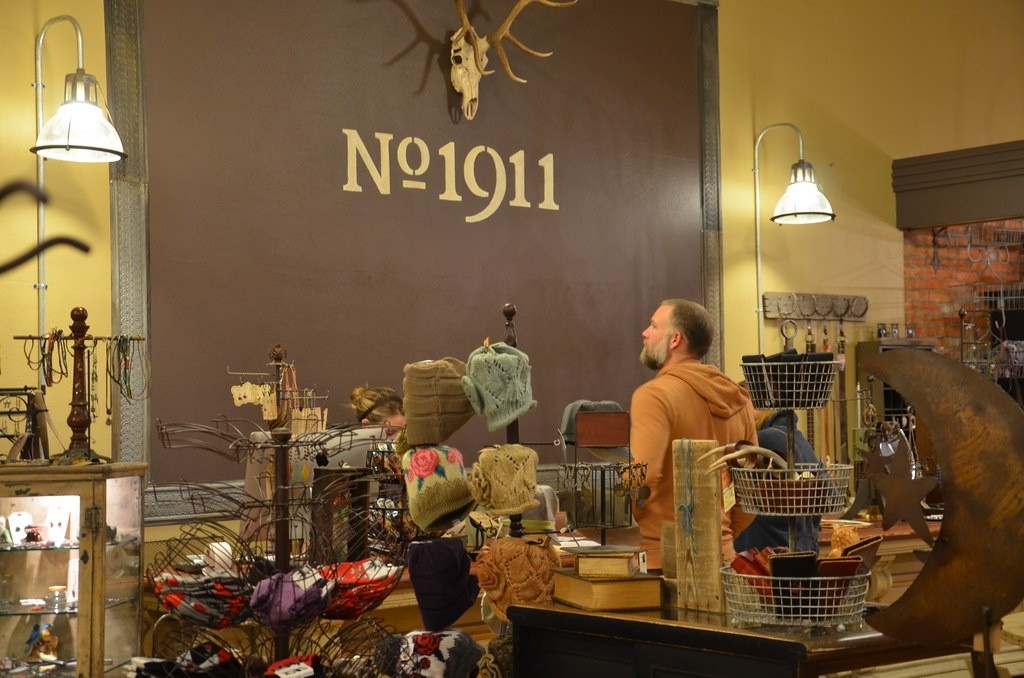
[468,444,541,515]
[397,628,485,678]
[481,591,513,639]
[475,635,513,678]
[402,444,479,533]
[460,337,537,432]
[476,537,562,623]
[407,535,480,631]
[403,357,477,447]
[559,400,635,465]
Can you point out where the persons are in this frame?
[622,298,759,575]
[351,385,405,440]
[733,380,840,619]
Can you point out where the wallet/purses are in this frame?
[730,536,884,619]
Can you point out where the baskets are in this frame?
[948,260,1024,311]
[720,566,872,627]
[949,318,1024,378]
[730,463,855,517]
[739,361,839,410]
[945,220,1024,246]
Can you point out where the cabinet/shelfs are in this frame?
[1,461,149,678]
[507,598,975,677]
[856,338,935,459]
[146,345,422,678]
[719,359,871,627]
[930,222,1024,406]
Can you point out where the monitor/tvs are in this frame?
[239,429,386,542]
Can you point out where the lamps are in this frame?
[754,121,836,354]
[30,14,127,389]
